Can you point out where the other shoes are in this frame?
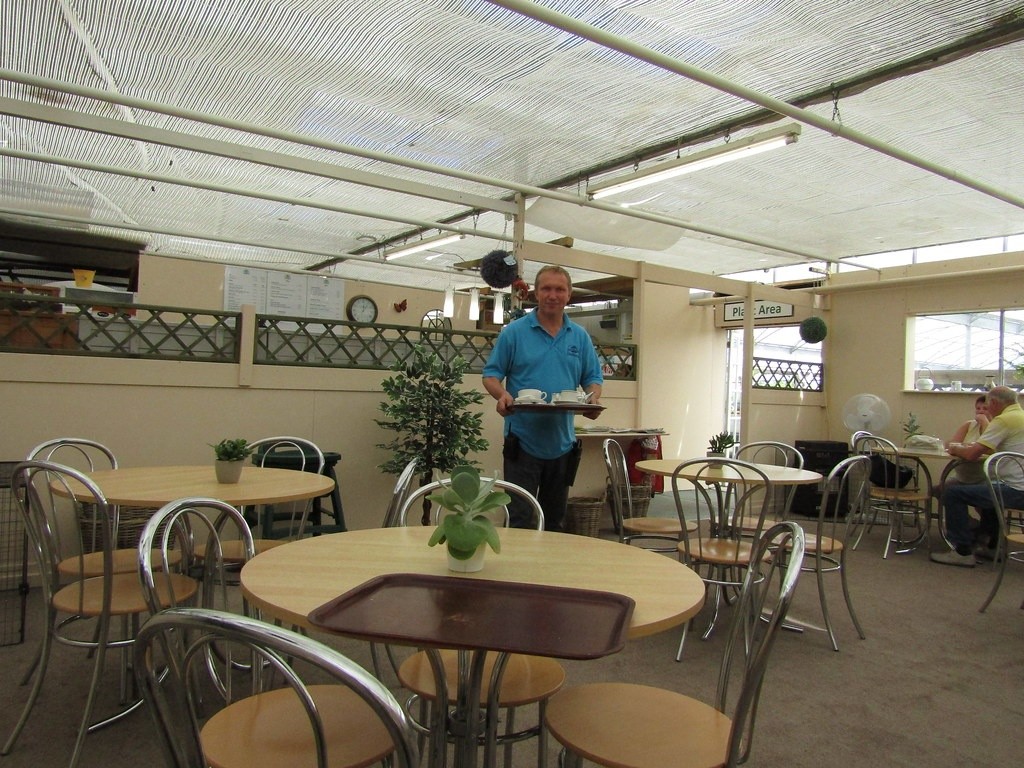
[974,546,1002,562]
[930,549,975,568]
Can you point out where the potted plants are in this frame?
[901,413,923,447]
[706,431,734,468]
[210,439,253,483]
[425,465,511,573]
[73,264,96,288]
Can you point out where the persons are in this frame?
[482,265,603,529]
[933,395,993,536]
[930,386,1024,567]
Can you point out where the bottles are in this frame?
[983,376,996,391]
[916,376,934,391]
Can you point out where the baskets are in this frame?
[605,475,651,535]
[76,500,177,553]
[566,492,607,538]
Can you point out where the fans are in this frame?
[841,392,891,451]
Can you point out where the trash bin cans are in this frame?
[790,440,848,517]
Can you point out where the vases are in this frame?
[982,375,996,392]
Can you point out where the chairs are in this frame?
[0,429,1024,768]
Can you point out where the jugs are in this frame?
[576,384,594,405]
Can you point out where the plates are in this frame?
[553,400,579,405]
[513,398,545,404]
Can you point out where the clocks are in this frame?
[346,295,378,328]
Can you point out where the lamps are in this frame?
[584,123,802,200]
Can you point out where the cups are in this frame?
[518,389,547,399]
[951,381,962,392]
[949,443,962,448]
[562,390,577,400]
[72,268,96,288]
[551,393,562,405]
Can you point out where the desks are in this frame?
[49,467,335,730]
[874,447,958,554]
[237,527,706,768]
[636,459,822,642]
